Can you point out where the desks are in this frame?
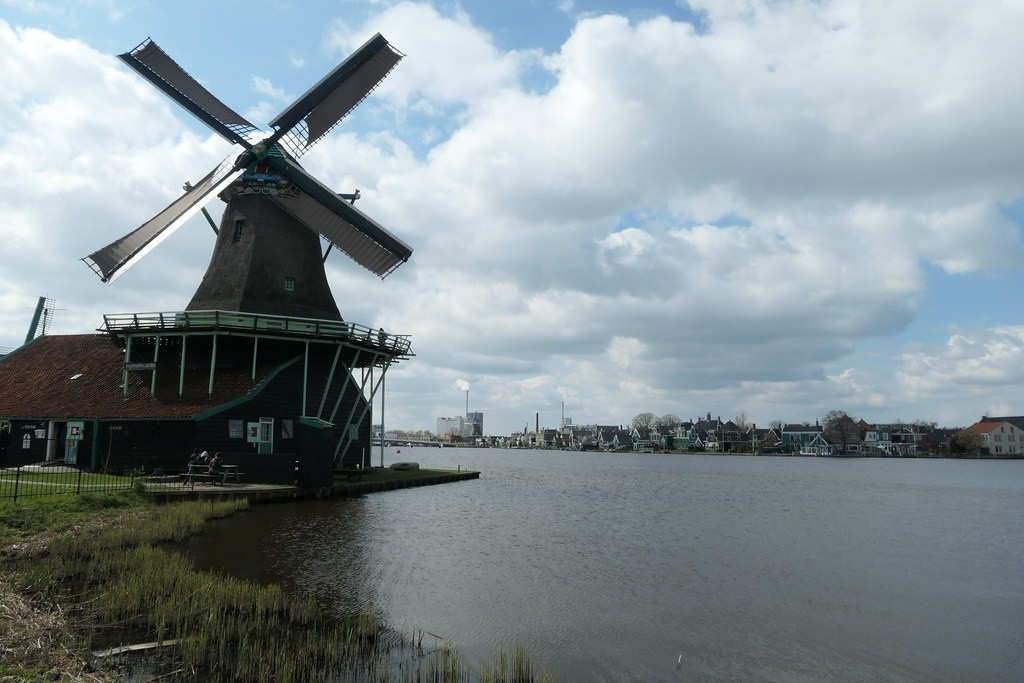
[182,464,241,485]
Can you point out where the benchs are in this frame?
[178,473,234,478]
[203,472,246,476]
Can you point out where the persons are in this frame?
[183,451,208,485]
[208,452,223,474]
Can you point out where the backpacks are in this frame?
[190,453,200,461]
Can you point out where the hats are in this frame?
[216,452,222,458]
